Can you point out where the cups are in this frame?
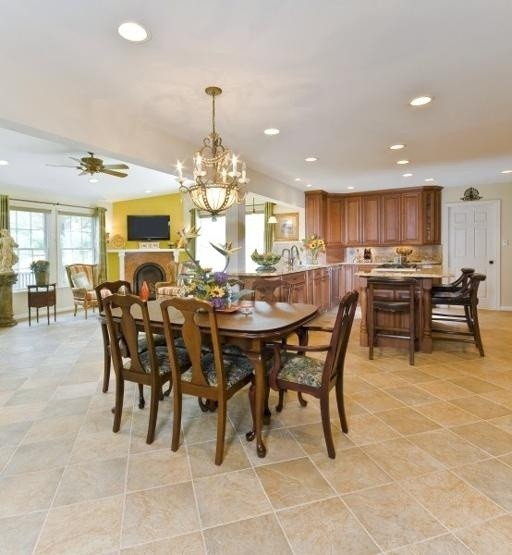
[239,290,254,313]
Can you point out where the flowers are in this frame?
[169,226,241,308]
[302,232,326,259]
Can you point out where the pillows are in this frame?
[72,273,92,293]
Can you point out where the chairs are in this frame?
[161,297,260,466]
[433,275,488,357]
[248,289,360,459]
[93,280,176,410]
[245,280,288,377]
[63,262,113,322]
[104,293,208,447]
[361,277,421,367]
[437,267,478,337]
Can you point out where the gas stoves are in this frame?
[372,264,422,271]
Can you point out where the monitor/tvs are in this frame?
[127,215,170,240]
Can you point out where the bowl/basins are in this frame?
[255,265,278,274]
[250,257,280,265]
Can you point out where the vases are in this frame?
[311,252,318,265]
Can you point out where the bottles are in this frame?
[141,279,149,301]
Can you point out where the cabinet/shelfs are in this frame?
[238,264,373,319]
[400,181,442,247]
[358,191,383,250]
[380,185,401,249]
[324,192,344,248]
[345,188,362,248]
[301,188,329,248]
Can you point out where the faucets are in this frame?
[281,248,290,265]
[291,245,300,266]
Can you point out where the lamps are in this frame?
[268,201,277,224]
[171,84,250,223]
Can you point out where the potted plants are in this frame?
[30,258,51,286]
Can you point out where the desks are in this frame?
[94,298,322,458]
[25,282,59,329]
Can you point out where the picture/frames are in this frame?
[271,212,300,241]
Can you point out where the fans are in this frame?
[42,151,134,180]
